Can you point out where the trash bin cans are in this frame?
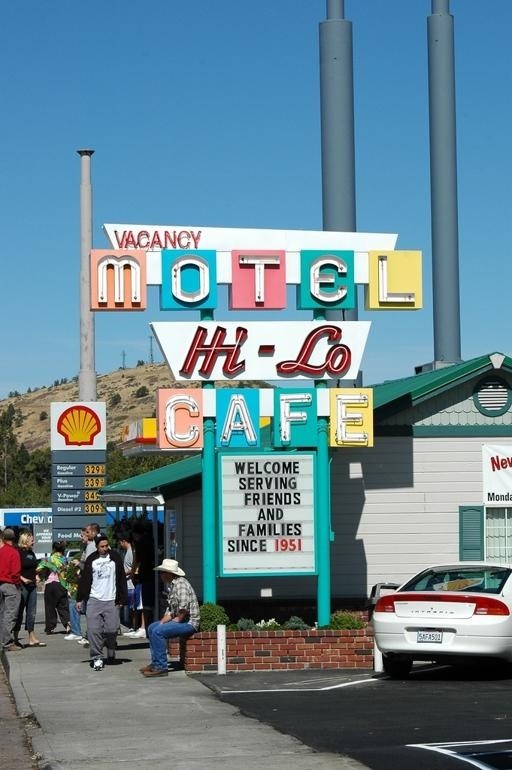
[367,581,401,607]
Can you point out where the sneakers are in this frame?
[140,663,168,677]
[3,642,23,651]
[107,647,115,662]
[77,637,89,645]
[64,633,83,641]
[122,627,147,639]
[94,658,103,671]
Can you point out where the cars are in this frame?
[371,559,511,678]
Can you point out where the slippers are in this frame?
[24,641,47,647]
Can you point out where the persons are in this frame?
[140,559,200,677]
[0,522,155,671]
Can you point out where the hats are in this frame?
[94,533,108,547]
[153,559,185,577]
